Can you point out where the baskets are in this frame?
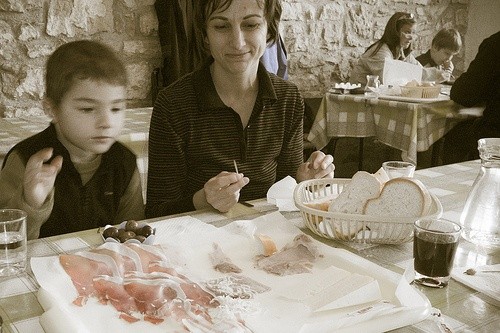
[400,86,442,99]
[293,178,444,245]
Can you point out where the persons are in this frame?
[145,0,335,214]
[349,12,463,95]
[442,31,499,165]
[0,39,144,242]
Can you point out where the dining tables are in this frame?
[0,107,152,206]
[0,159,500,333]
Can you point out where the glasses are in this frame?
[400,31,417,39]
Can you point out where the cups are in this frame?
[0,208,29,278]
[413,216,462,288]
[382,160,416,182]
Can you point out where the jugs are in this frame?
[364,74,381,98]
[458,137,500,251]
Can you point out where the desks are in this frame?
[308,93,485,171]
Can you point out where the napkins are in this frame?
[266,176,343,212]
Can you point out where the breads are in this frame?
[303,170,430,240]
